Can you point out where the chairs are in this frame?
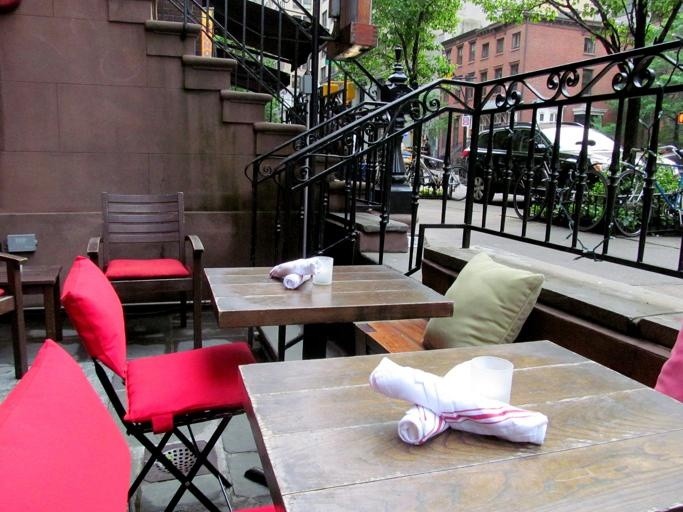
[0,252,28,380]
[59,256,257,512]
[1,338,285,512]
[86,191,205,350]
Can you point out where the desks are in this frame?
[0,264,63,342]
[203,265,454,488]
[237,338,683,512]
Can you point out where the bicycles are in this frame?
[611,145,683,238]
[404,145,469,201]
[512,137,610,231]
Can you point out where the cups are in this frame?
[471,355,514,404]
[311,255,334,285]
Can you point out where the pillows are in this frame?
[420,252,545,350]
[654,318,683,403]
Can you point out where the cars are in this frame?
[399,149,413,168]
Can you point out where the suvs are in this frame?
[457,120,680,219]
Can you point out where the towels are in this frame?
[269,258,322,289]
[369,355,548,446]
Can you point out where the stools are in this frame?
[352,318,430,356]
